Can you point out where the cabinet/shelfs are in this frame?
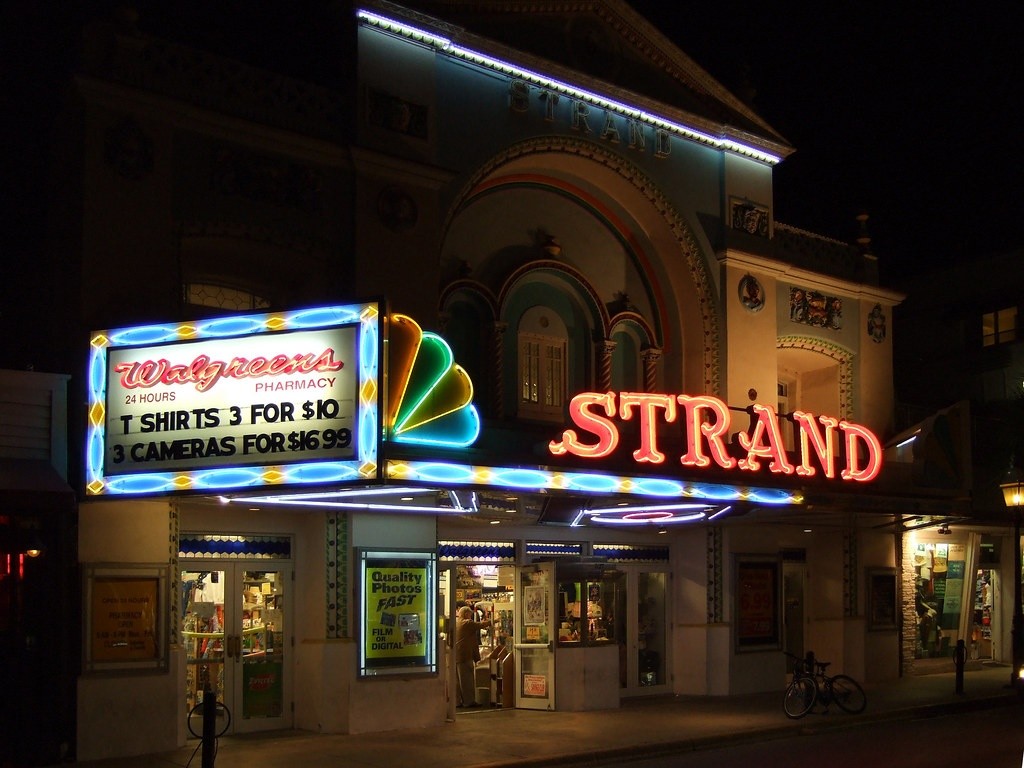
[182,602,282,715]
[456,587,514,707]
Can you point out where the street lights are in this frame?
[998,454,1023,687]
[7,516,42,644]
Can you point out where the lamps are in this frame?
[938,524,953,535]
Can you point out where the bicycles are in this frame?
[781,650,867,718]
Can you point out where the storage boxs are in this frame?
[497,565,514,587]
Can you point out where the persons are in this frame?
[456,606,500,708]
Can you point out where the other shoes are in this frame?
[464,700,483,709]
